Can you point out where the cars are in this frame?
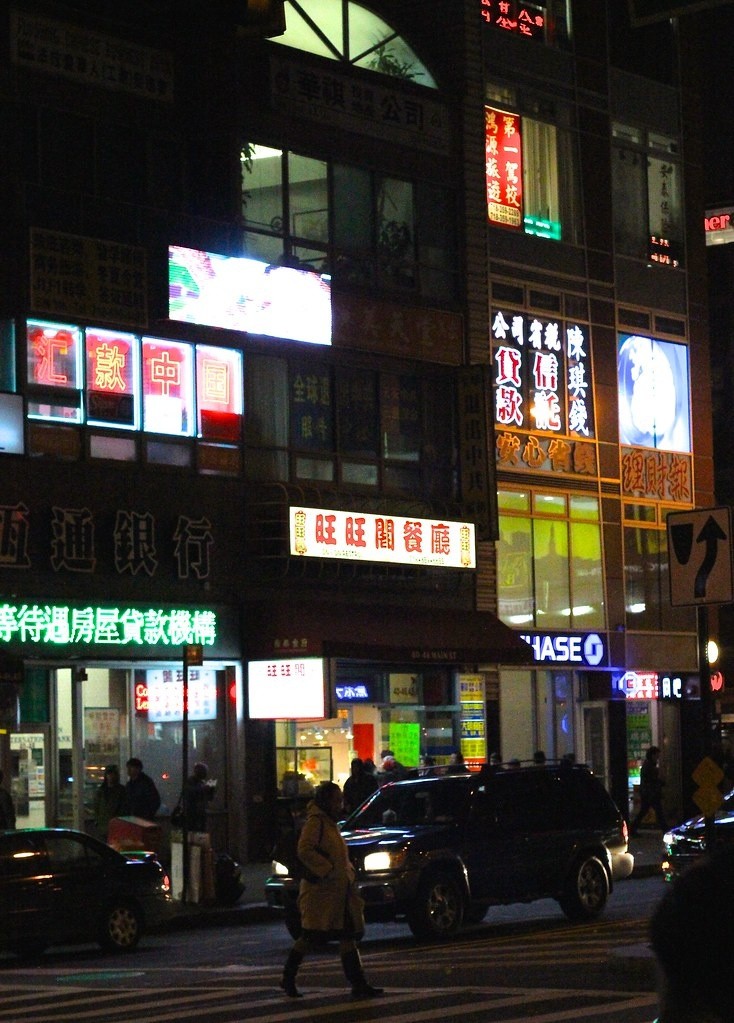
[0,827,176,952]
[662,789,734,884]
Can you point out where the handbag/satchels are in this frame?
[289,814,330,884]
[170,804,185,825]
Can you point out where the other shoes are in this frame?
[630,821,638,834]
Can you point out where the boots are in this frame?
[279,948,303,997]
[340,947,386,997]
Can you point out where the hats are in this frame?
[489,752,501,762]
[106,764,117,771]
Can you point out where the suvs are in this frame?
[265,759,634,942]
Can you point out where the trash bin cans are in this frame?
[107,816,158,860]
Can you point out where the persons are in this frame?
[279,782,385,998]
[180,763,217,906]
[343,749,578,814]
[94,764,125,841]
[628,746,672,834]
[122,759,162,821]
